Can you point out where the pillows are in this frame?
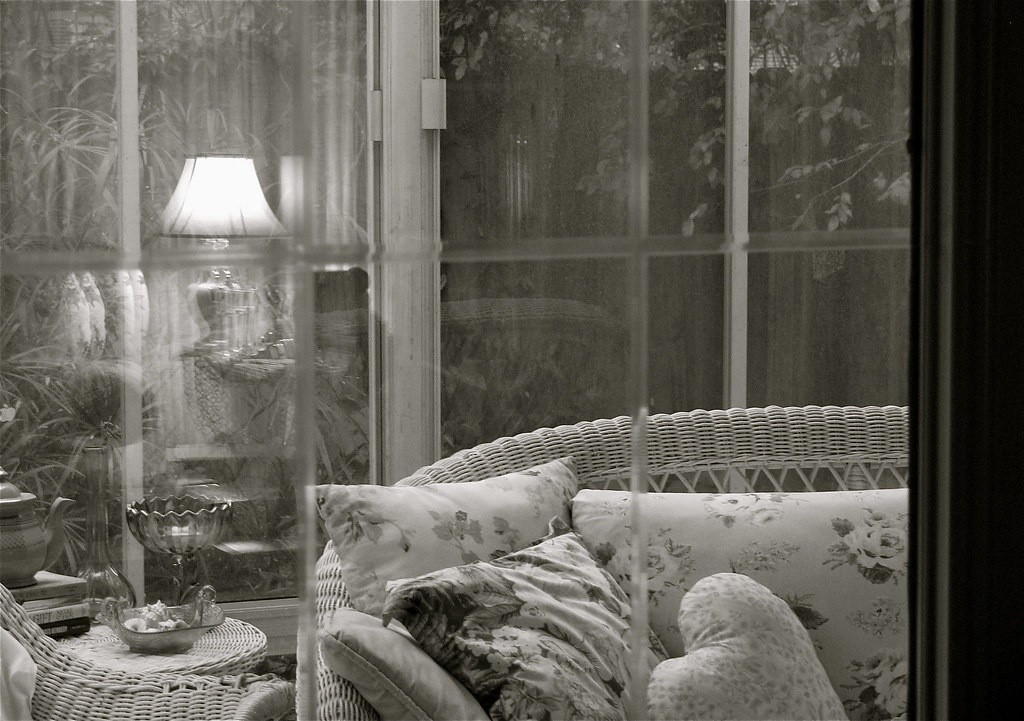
[318,607,491,721]
[306,456,580,617]
[646,573,849,721]
[571,488,909,721]
[380,514,670,721]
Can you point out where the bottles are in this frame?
[70,445,136,623]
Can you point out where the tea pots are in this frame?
[0,466,76,588]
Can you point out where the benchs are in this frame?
[295,403,913,721]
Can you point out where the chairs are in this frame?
[0,583,294,721]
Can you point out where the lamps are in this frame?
[149,153,291,348]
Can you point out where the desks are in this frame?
[54,618,269,678]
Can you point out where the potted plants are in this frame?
[0,64,178,366]
